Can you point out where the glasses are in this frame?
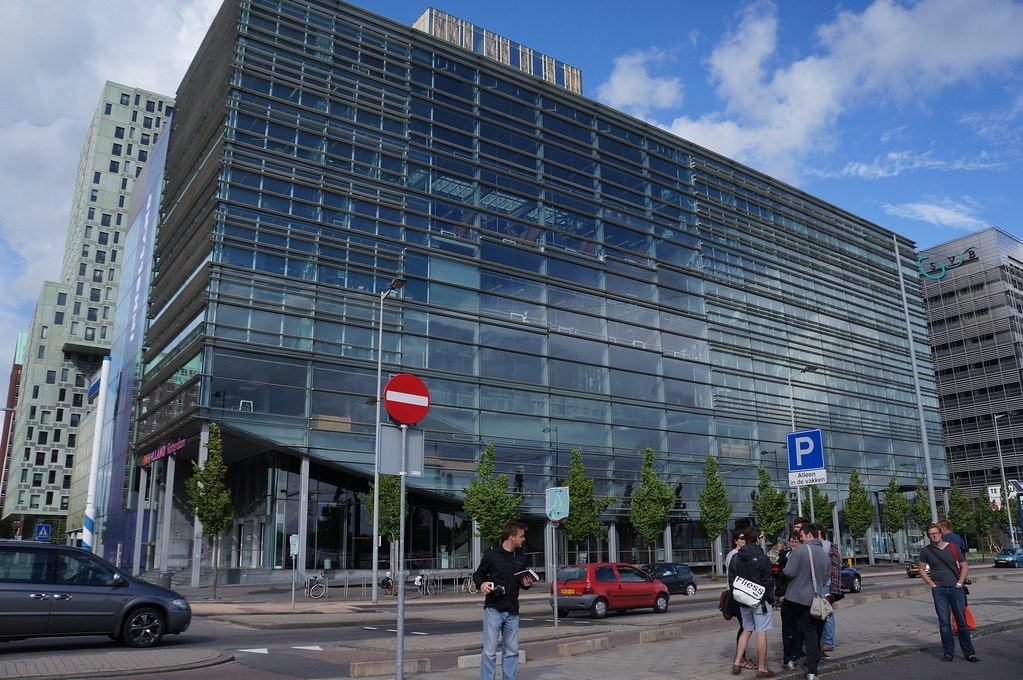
[929,532,940,535]
[738,536,744,540]
[759,535,763,539]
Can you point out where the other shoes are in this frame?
[819,652,831,660]
[967,655,981,664]
[820,644,833,652]
[942,654,953,663]
[807,673,820,680]
[757,669,775,679]
[741,658,758,669]
[732,665,741,674]
[783,660,795,670]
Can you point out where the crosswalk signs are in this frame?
[36,524,51,540]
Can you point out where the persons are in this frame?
[794,517,809,532]
[779,523,832,680]
[778,530,803,604]
[936,520,966,562]
[918,524,981,663]
[815,523,844,652]
[728,526,777,678]
[472,522,532,680]
[724,530,758,669]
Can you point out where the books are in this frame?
[514,569,540,583]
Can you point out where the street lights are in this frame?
[371,275,405,603]
[788,365,817,517]
[0,407,15,498]
[994,410,1020,547]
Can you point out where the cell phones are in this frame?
[489,582,494,589]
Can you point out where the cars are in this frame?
[630,562,697,596]
[840,561,861,593]
[906,557,930,578]
[550,562,670,620]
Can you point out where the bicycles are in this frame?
[461,569,478,594]
[305,574,325,598]
[417,570,436,596]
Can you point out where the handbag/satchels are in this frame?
[718,590,733,620]
[962,585,969,607]
[733,576,766,608]
[810,594,833,621]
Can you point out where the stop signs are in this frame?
[383,373,430,425]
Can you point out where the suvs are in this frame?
[994,548,1023,568]
[0,539,191,648]
